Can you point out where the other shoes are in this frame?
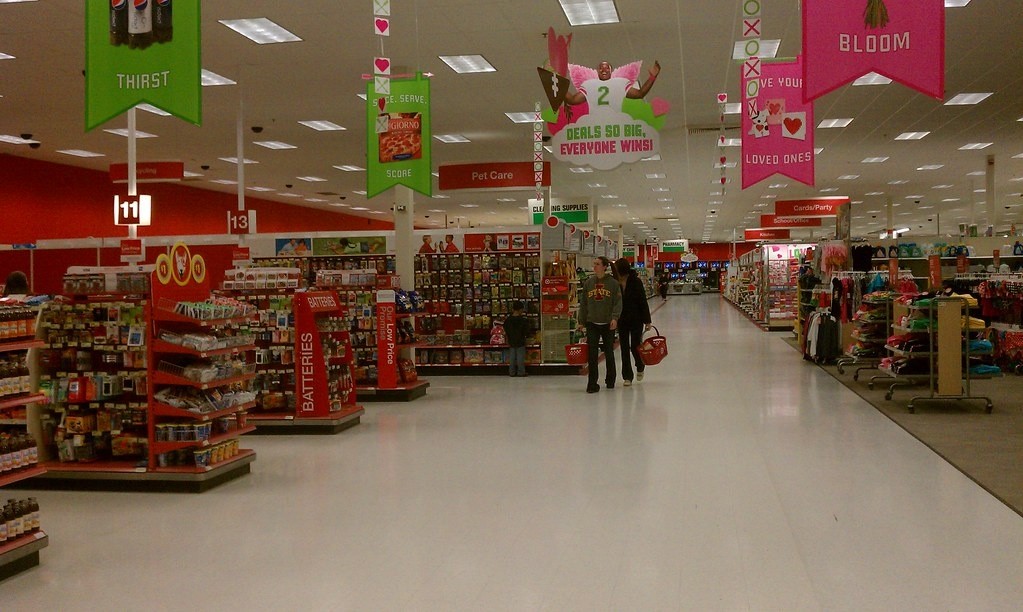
[606,382,614,388]
[624,380,632,386]
[636,370,644,382]
[588,384,600,393]
[509,371,528,377]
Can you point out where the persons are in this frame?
[483,234,495,252]
[282,240,298,254]
[614,257,652,385]
[498,239,507,248]
[419,235,437,253]
[658,272,669,301]
[294,239,306,254]
[579,256,624,393]
[439,235,459,252]
[3,271,31,297]
[327,238,384,255]
[564,59,661,116]
[798,266,821,312]
[503,300,530,377]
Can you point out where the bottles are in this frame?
[0,432,39,476]
[322,334,336,356]
[0,352,30,402]
[0,300,35,343]
[315,317,348,332]
[0,497,40,546]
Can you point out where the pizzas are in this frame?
[380,133,421,162]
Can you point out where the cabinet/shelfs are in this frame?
[543,251,612,368]
[153,295,257,481]
[724,241,1002,380]
[377,289,429,392]
[0,294,49,566]
[295,292,364,425]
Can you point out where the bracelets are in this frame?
[650,75,656,81]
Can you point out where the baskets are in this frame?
[564,329,588,366]
[637,326,668,365]
